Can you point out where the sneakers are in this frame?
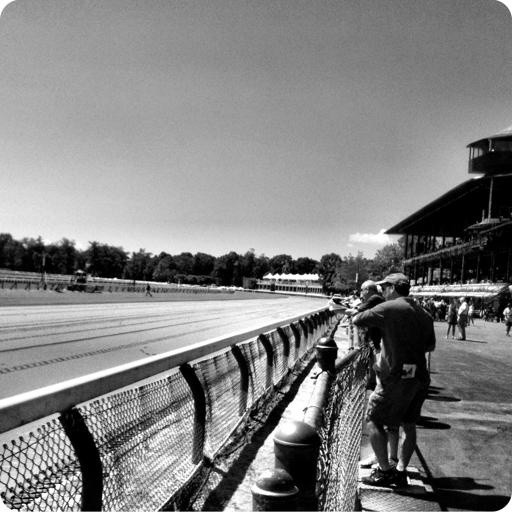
[361,467,397,485]
[359,453,381,466]
[445,335,466,341]
[370,458,399,470]
[393,468,408,489]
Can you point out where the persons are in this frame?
[144,283,152,298]
[340,270,511,490]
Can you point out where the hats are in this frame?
[361,280,377,290]
[458,296,465,301]
[375,272,412,289]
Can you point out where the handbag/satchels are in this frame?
[459,314,467,327]
